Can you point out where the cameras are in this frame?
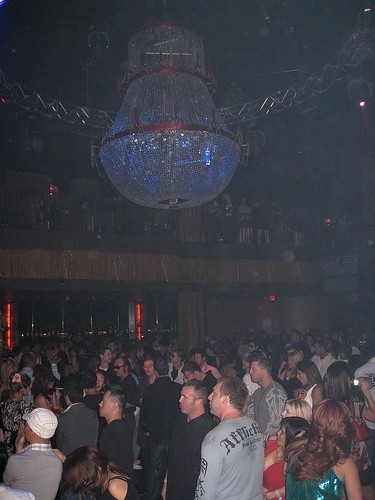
[353,379,360,386]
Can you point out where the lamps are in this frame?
[97,1,243,210]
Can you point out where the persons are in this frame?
[52,446,140,500]
[4,407,63,500]
[0,328,221,462]
[141,357,183,500]
[160,378,220,500]
[208,192,283,244]
[221,325,375,500]
[194,376,264,500]
[99,389,136,486]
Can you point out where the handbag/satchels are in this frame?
[350,399,371,442]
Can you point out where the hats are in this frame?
[22,408,58,439]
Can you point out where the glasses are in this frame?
[248,344,255,348]
[171,353,177,357]
[114,365,125,369]
[107,345,115,347]
